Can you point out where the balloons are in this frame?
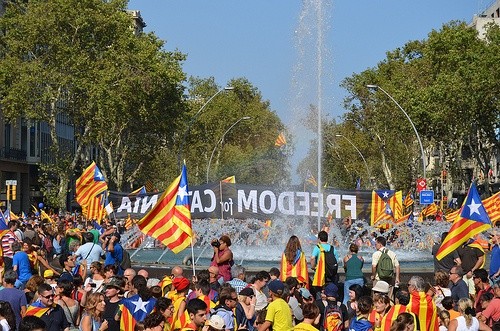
[38,203,45,209]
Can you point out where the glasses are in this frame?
[40,294,55,299]
[249,295,255,299]
[491,235,495,237]
[450,272,458,275]
[473,277,479,279]
[229,297,238,301]
[90,267,94,269]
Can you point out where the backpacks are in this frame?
[120,245,131,270]
[322,300,343,331]
[317,245,338,277]
[376,248,394,280]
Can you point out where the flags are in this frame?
[356,178,361,190]
[305,169,318,187]
[275,132,288,156]
[418,202,461,223]
[137,159,192,254]
[125,215,133,231]
[435,181,493,261]
[221,175,236,184]
[132,186,147,194]
[371,189,414,231]
[444,191,500,226]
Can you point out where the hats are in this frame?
[239,288,254,296]
[172,277,190,291]
[325,284,339,297]
[267,280,284,295]
[205,315,225,330]
[102,276,125,295]
[372,281,389,293]
[82,232,94,241]
[44,270,53,277]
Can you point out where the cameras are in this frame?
[91,284,96,287]
[211,239,220,247]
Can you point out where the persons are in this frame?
[195,218,401,249]
[457,238,484,294]
[311,230,340,284]
[343,244,364,304]
[279,235,309,289]
[489,227,500,284]
[0,252,500,331]
[76,161,113,235]
[432,232,462,275]
[371,237,400,288]
[0,206,137,275]
[210,236,234,283]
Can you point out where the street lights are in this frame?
[366,84,426,179]
[206,117,251,184]
[345,118,385,167]
[335,134,370,176]
[178,87,235,177]
